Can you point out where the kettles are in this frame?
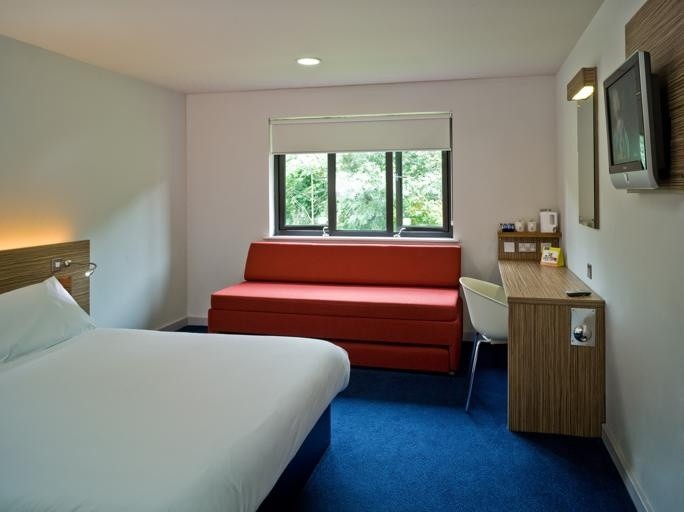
[539,209,558,234]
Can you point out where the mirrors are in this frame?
[574,95,598,231]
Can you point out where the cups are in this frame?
[527,222,537,233]
[514,221,525,233]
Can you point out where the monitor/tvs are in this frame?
[603,48,660,190]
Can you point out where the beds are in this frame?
[0,233,349,512]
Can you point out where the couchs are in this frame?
[202,239,464,378]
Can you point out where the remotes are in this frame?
[566,290,593,296]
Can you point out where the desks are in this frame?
[495,259,607,442]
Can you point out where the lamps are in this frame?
[50,254,98,280]
[565,65,594,104]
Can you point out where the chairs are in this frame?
[456,273,507,415]
